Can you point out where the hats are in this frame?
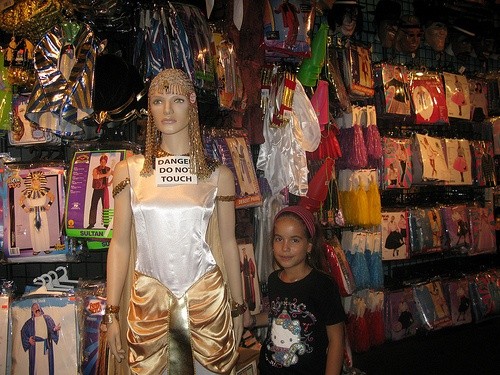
[274,206,316,237]
[100,156,108,163]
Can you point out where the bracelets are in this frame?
[105,314,119,323]
[106,305,120,314]
[249,314,256,329]
[231,301,247,318]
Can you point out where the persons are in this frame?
[18,170,56,255]
[243,206,347,375]
[420,137,437,176]
[385,67,410,115]
[451,207,468,245]
[470,83,486,122]
[453,141,469,182]
[397,296,414,335]
[385,214,406,256]
[388,164,397,185]
[87,156,114,229]
[456,282,470,321]
[452,76,467,116]
[358,47,368,85]
[431,209,440,245]
[21,303,62,375]
[104,68,247,375]
[399,140,407,186]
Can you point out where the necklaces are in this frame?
[153,146,190,158]
[280,272,298,283]
